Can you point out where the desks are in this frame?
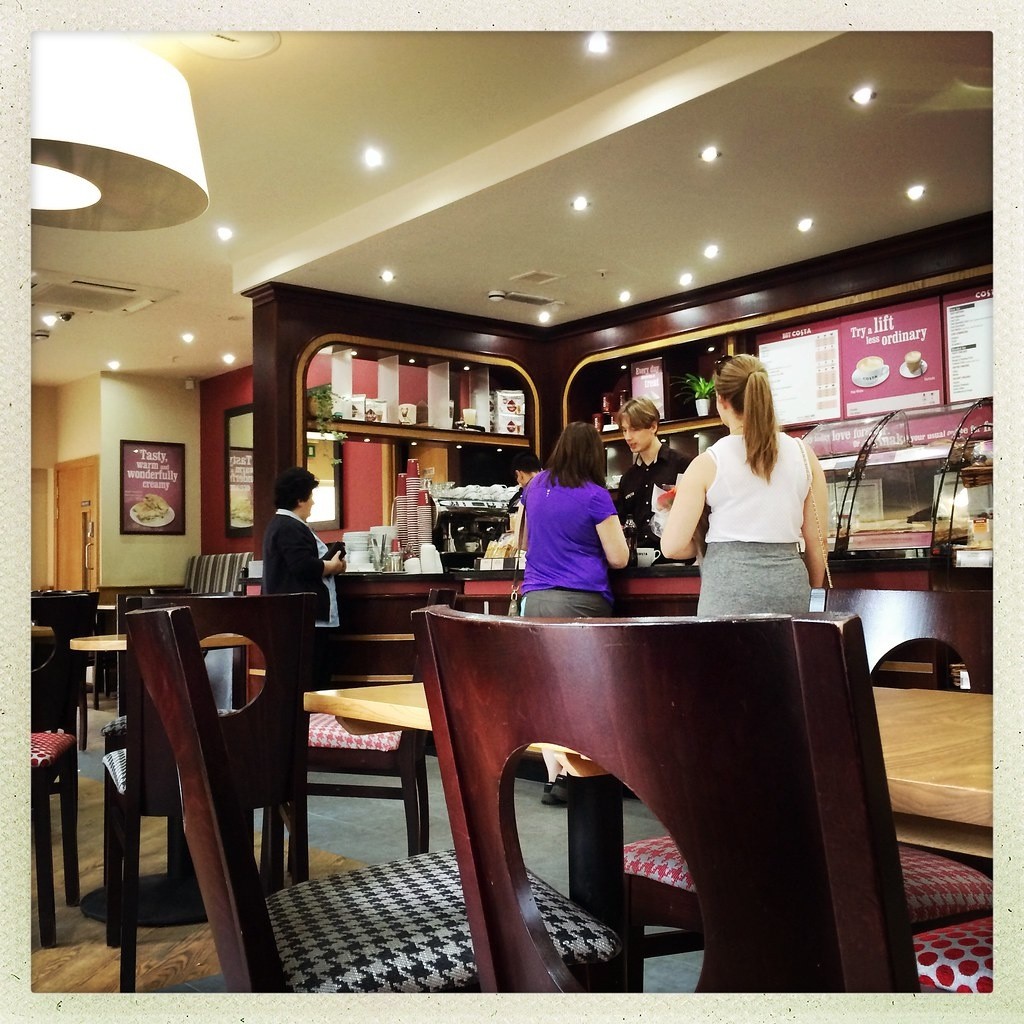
[87,605,115,709]
[32,625,88,751]
[304,681,993,992]
[69,630,334,928]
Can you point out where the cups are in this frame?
[904,350,922,373]
[856,356,889,384]
[465,542,479,552]
[452,484,518,501]
[391,459,444,574]
[463,408,477,426]
[373,546,392,572]
[636,547,661,567]
[389,552,403,571]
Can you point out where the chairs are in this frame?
[31,552,993,992]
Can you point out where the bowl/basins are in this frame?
[342,525,395,570]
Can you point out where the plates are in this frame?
[130,503,175,527]
[851,369,890,388]
[899,359,927,378]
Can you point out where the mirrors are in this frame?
[222,383,340,538]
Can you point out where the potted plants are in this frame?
[304,384,344,426]
[667,370,714,417]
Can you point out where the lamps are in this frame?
[32,31,210,232]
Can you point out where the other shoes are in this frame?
[551,774,569,804]
[541,782,556,805]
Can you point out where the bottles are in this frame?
[624,514,638,568]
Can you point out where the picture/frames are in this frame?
[225,446,255,532]
[120,440,186,535]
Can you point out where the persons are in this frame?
[263,467,346,691]
[521,421,630,806]
[508,450,543,533]
[661,354,828,617]
[616,398,694,564]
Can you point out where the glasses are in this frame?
[715,355,733,376]
